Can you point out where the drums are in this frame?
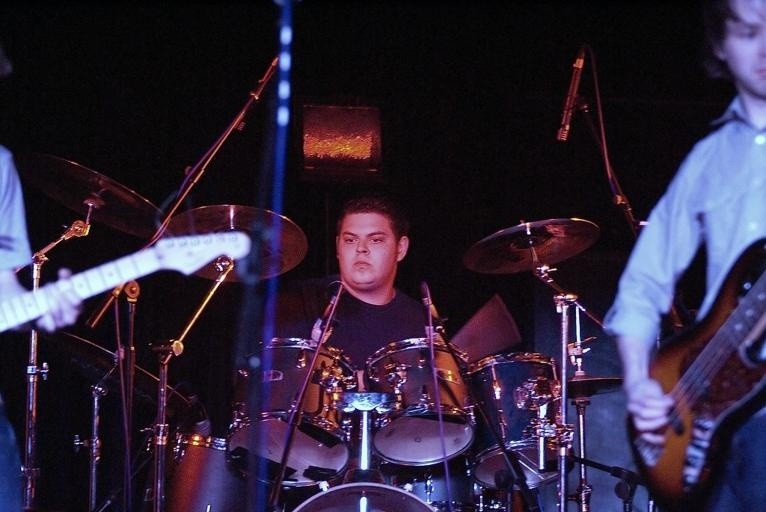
[467,352,579,490]
[168,434,259,512]
[228,337,359,481]
[363,340,474,467]
[292,482,437,511]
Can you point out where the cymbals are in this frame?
[159,205,308,282]
[568,330,625,396]
[464,219,602,273]
[54,326,192,417]
[12,150,164,237]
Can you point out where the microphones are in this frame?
[311,295,339,343]
[187,394,212,436]
[422,297,446,340]
[235,57,279,132]
[556,52,585,141]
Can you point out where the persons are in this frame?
[1,144,82,512]
[609,0,766,512]
[260,188,447,365]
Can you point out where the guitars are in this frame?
[623,237,766,510]
